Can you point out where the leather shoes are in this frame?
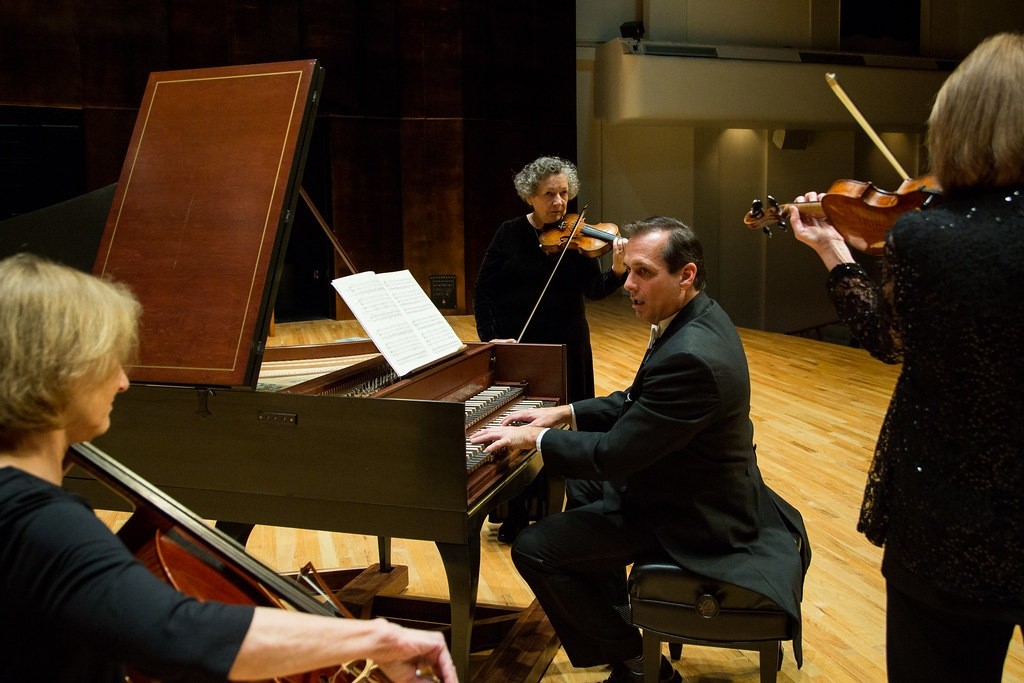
[597,655,682,683]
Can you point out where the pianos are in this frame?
[0,53,576,683]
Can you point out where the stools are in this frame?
[627,508,805,683]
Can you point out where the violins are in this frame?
[744,171,945,258]
[537,212,630,259]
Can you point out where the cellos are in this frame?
[63,442,383,682]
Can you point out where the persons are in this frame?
[0,254,459,683]
[466,157,628,541]
[789,33,1024,682]
[471,216,812,683]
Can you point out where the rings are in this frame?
[617,249,625,254]
